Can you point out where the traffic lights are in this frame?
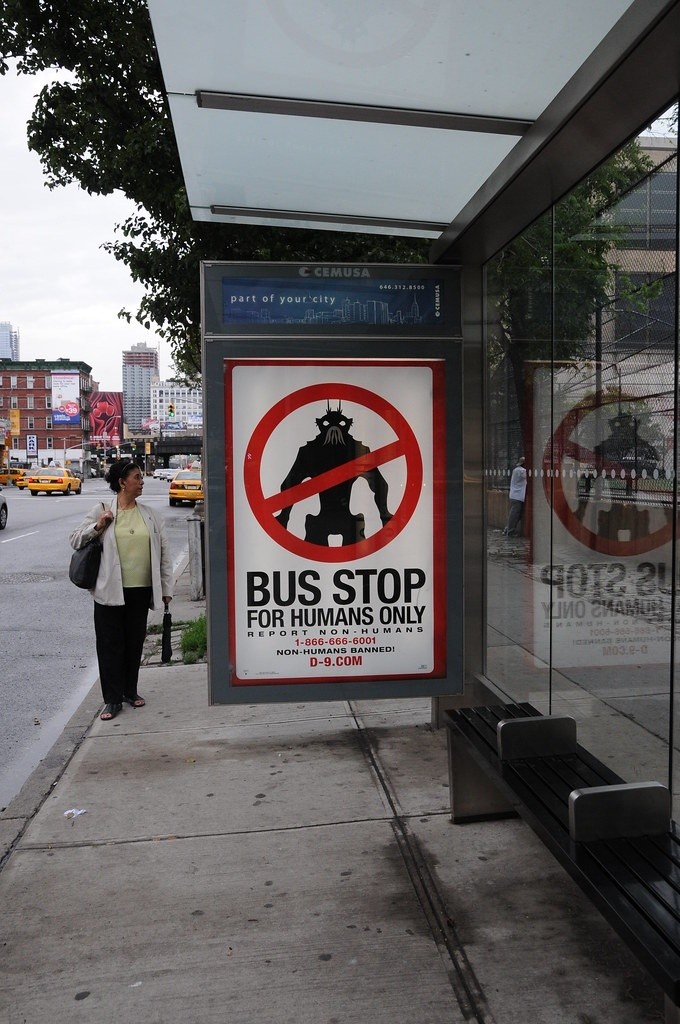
[168,403,176,417]
[132,444,136,451]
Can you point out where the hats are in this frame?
[515,456,525,467]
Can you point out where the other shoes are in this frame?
[502,530,507,535]
[507,532,519,537]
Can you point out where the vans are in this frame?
[0,468,30,487]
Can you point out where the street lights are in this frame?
[8,378,35,431]
[104,415,121,473]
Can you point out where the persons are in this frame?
[502,456,529,539]
[68,459,173,723]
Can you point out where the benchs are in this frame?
[442,703,680,1007]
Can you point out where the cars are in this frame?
[27,460,82,496]
[15,465,38,490]
[70,468,84,483]
[168,462,204,507]
[0,486,8,530]
[153,468,182,482]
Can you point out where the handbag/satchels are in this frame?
[68,501,107,589]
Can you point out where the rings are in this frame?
[105,513,109,515]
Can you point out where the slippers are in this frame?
[99,701,123,720]
[122,693,145,707]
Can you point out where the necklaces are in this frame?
[118,499,136,534]
